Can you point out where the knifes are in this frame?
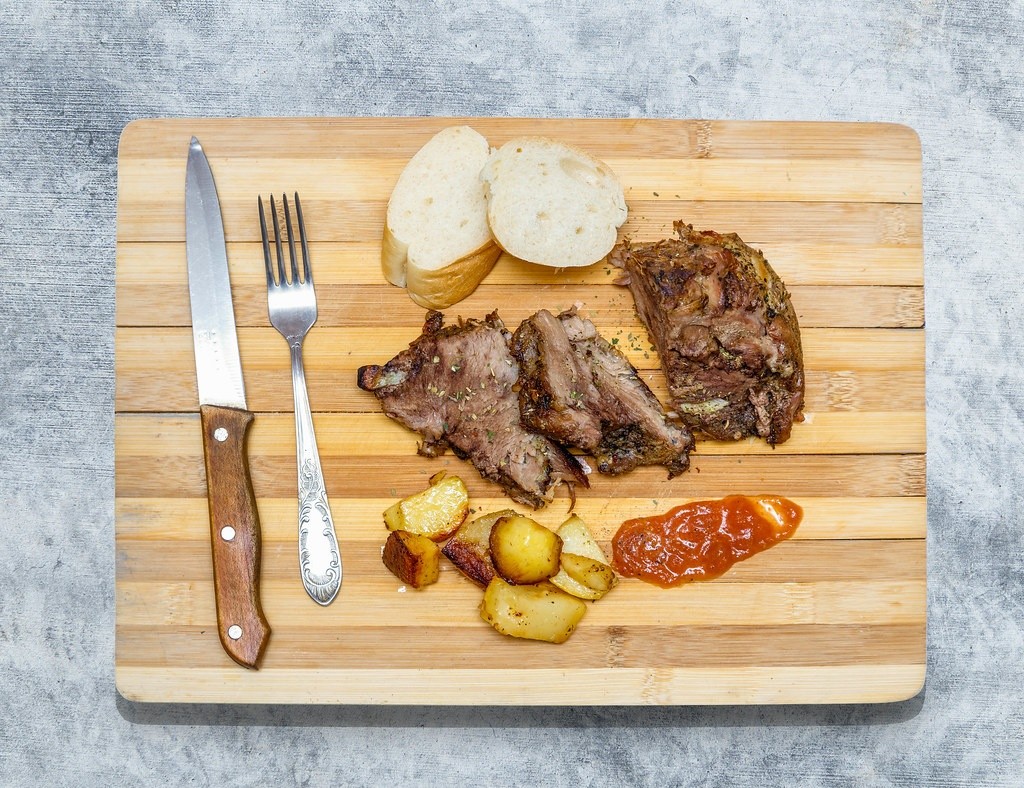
[185,135,273,671]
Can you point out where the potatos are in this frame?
[381,478,620,645]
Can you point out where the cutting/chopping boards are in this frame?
[116,117,928,706]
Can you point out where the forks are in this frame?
[257,191,343,607]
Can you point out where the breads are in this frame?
[480,136,627,268]
[382,126,502,311]
